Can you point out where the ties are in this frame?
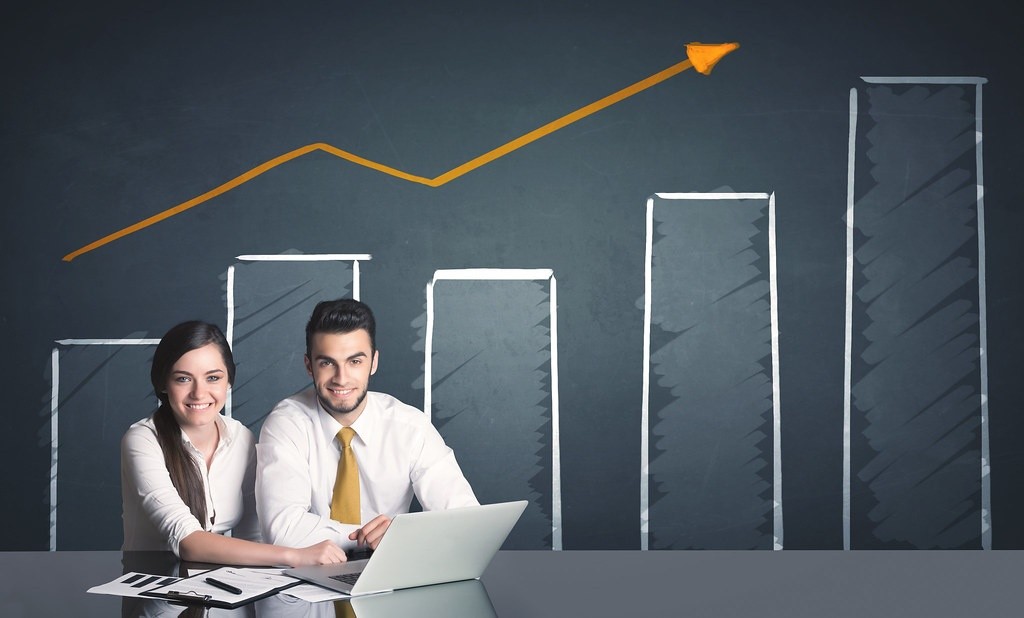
[330,427,361,525]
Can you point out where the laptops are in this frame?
[281,501,529,596]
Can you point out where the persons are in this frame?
[121,321,347,566]
[255,299,480,563]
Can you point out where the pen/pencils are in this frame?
[207,577,244,595]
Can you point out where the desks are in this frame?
[0,551,1024,618]
